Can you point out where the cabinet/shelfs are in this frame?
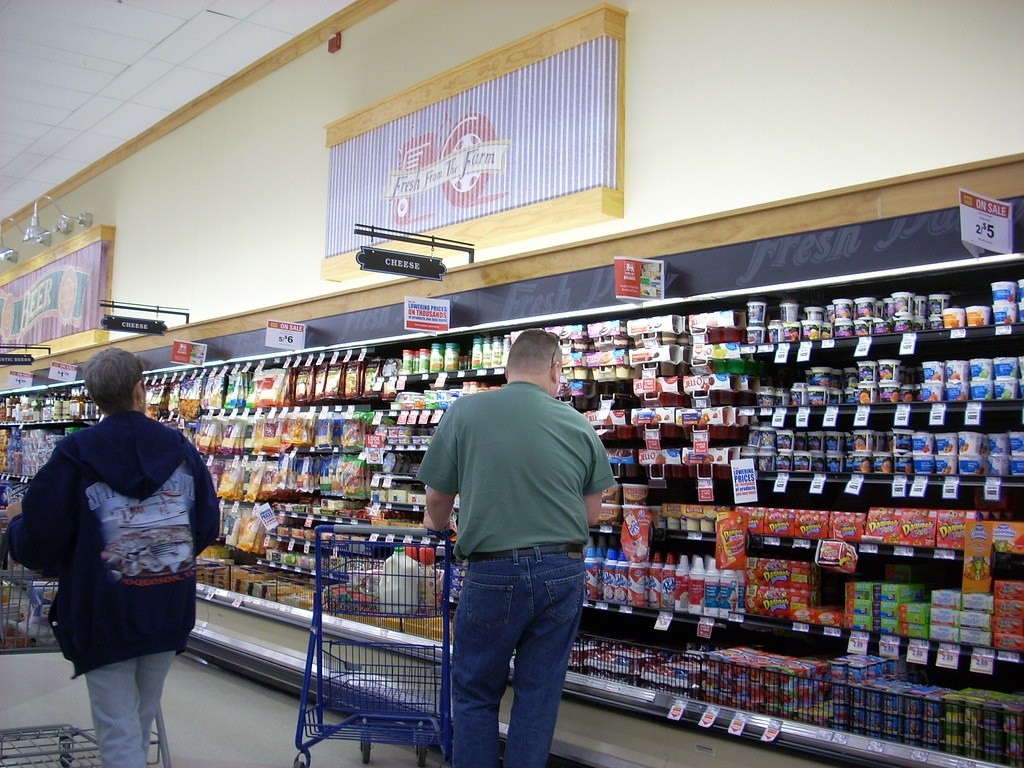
[0,249,1024,768]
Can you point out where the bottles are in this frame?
[0,386,101,422]
[378,548,419,613]
[402,334,512,375]
[583,536,746,619]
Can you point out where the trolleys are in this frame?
[1,509,172,767]
[291,524,455,767]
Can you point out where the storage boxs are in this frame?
[197,549,453,642]
[568,560,1023,768]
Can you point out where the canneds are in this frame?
[402,342,461,374]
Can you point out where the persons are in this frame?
[416,329,615,768]
[6,347,221,768]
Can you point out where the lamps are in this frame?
[0,195,93,263]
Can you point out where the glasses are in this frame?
[548,332,561,368]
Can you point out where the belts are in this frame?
[470,544,584,562]
